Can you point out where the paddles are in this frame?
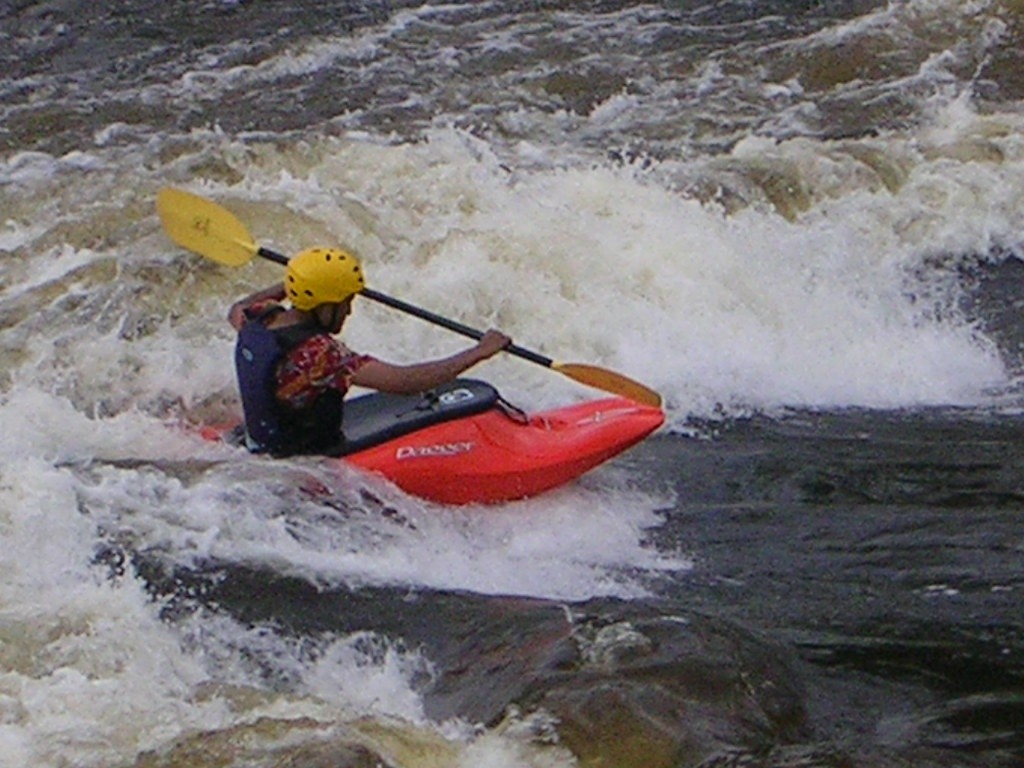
[154,174,661,413]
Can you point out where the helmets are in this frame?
[283,247,365,313]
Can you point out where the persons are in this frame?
[226,246,514,460]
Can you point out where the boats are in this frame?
[198,375,666,510]
